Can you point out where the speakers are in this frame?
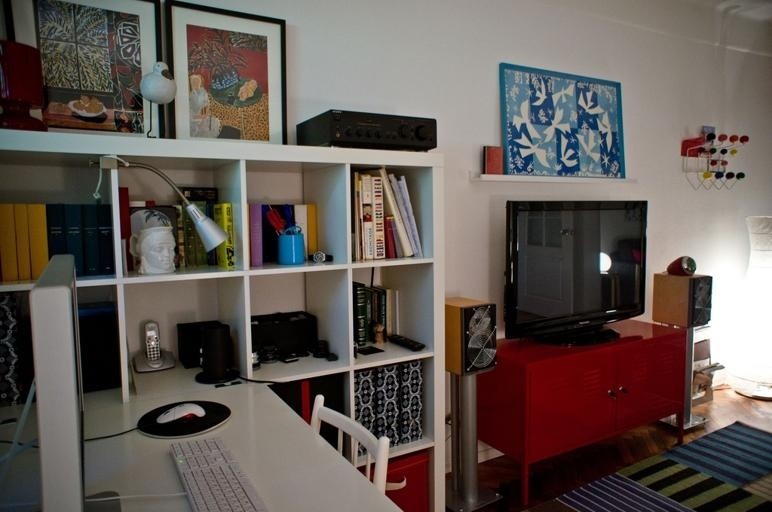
[192,322,240,384]
[445,297,496,377]
[652,271,712,328]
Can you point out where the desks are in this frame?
[0,386,403,512]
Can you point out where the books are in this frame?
[118,188,236,271]
[1,203,116,281]
[246,203,317,266]
[352,168,423,347]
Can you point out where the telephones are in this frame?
[132,321,175,372]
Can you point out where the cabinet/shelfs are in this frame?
[0,130,446,512]
[478,316,687,512]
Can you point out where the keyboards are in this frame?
[169,436,270,512]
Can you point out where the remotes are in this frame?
[389,334,426,352]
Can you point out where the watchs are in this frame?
[309,252,333,262]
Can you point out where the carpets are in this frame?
[536,422,772,512]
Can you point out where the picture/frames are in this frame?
[164,0,287,144]
[499,63,628,179]
[5,0,165,139]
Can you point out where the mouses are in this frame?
[156,402,206,424]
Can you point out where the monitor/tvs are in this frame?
[505,201,647,345]
[0,253,122,512]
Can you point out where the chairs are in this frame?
[311,394,391,495]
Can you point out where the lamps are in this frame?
[724,215,772,401]
[92,152,229,253]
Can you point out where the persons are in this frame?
[135,226,177,276]
